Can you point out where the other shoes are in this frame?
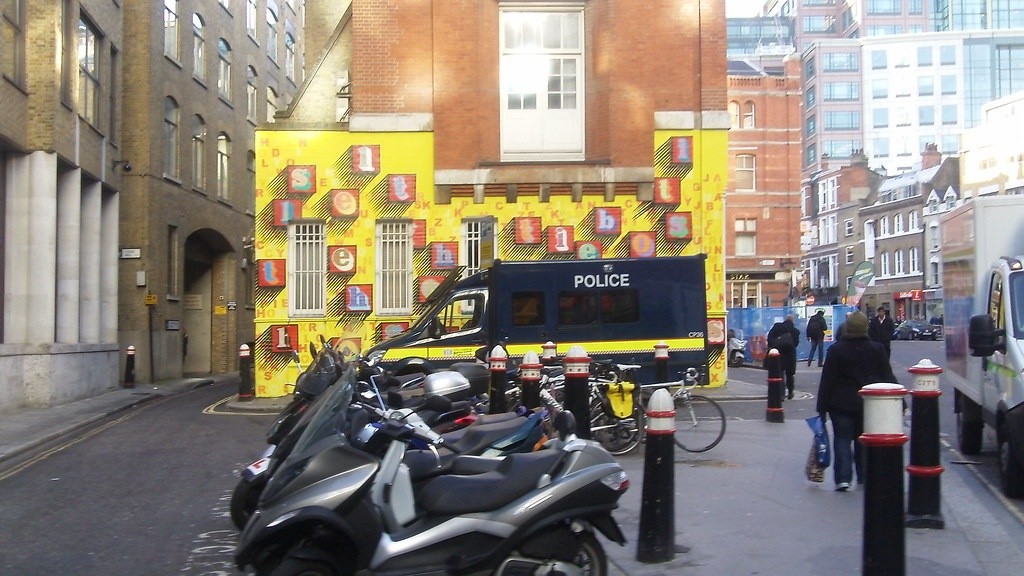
[788,393,794,399]
[818,365,823,367]
[833,482,849,491]
[808,359,811,367]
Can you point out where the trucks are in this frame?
[939,195,1024,499]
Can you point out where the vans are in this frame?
[359,253,710,402]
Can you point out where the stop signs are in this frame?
[806,297,814,305]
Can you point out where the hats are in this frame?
[844,309,867,337]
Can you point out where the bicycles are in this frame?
[502,359,727,457]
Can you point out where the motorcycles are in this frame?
[727,328,748,366]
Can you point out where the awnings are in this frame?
[806,296,838,305]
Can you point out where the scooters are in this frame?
[230,332,632,576]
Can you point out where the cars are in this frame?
[895,321,937,340]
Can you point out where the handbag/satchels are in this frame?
[805,414,830,482]
[776,333,795,349]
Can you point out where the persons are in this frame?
[817,312,908,492]
[767,314,800,400]
[806,310,827,367]
[835,312,852,341]
[867,306,894,360]
[930,314,943,325]
